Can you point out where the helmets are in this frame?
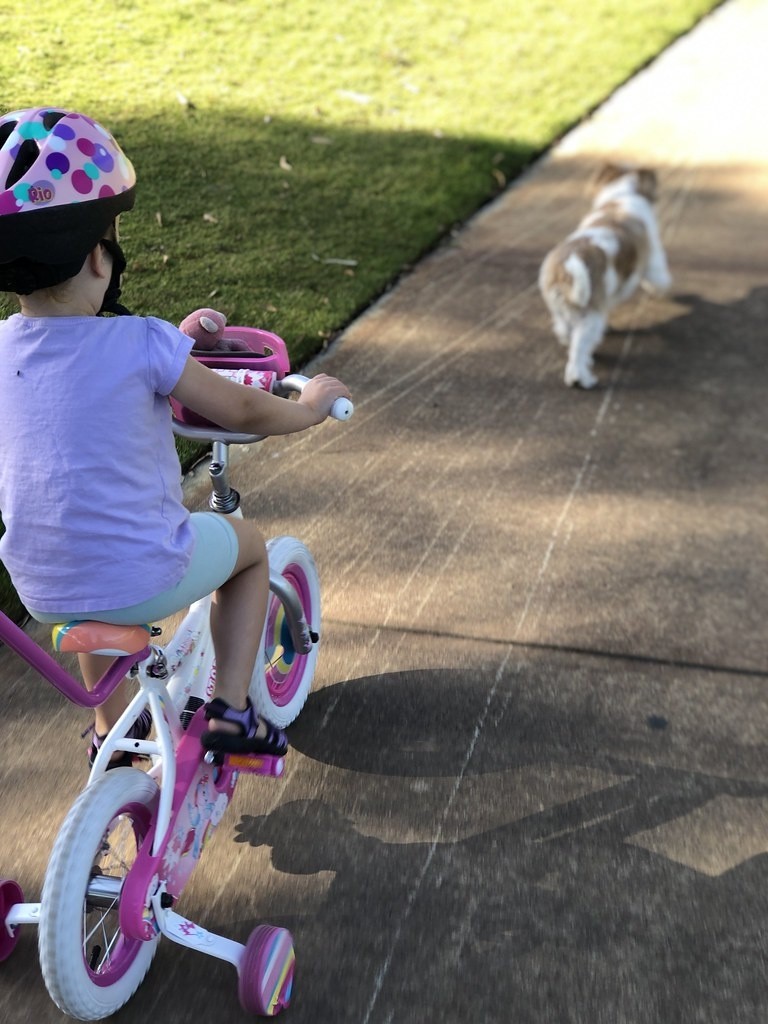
[0,106,137,295]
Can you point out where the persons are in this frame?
[0,108,352,777]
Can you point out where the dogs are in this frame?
[536,161,673,390]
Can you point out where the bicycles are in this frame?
[1,308,352,1023]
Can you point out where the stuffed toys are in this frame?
[178,308,258,354]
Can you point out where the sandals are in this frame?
[82,707,153,775]
[201,695,288,757]
